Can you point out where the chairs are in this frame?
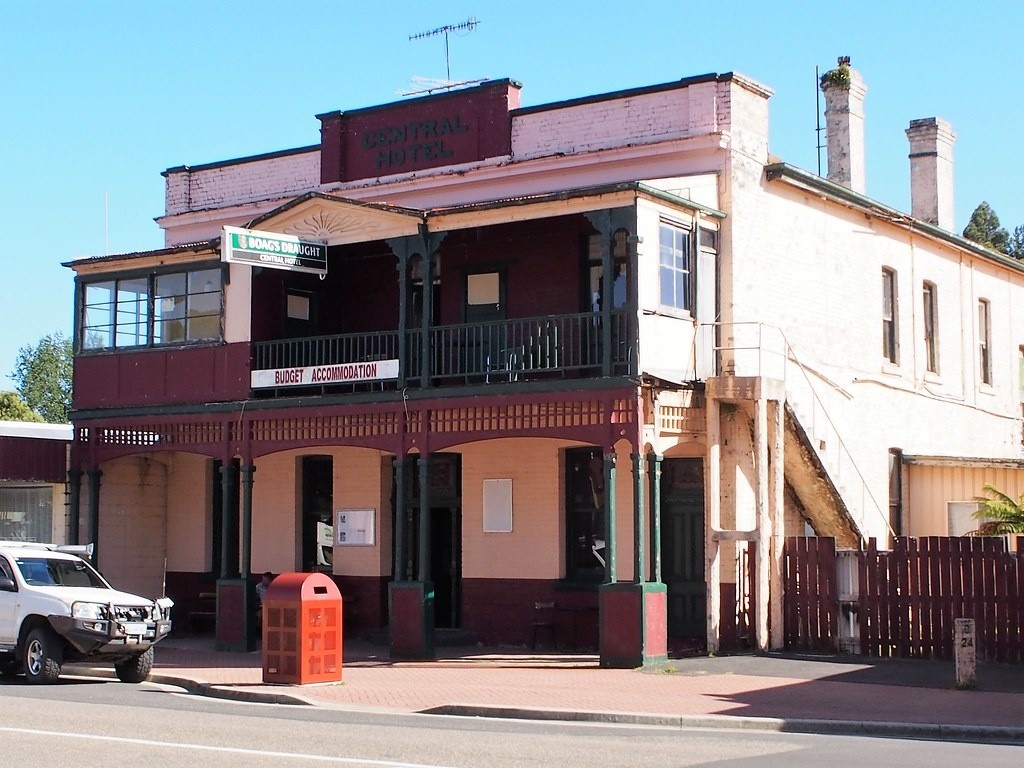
[532,601,556,650]
[485,347,524,382]
[352,353,388,393]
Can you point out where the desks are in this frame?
[554,606,600,653]
[199,593,217,599]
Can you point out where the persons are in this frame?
[255,572,274,626]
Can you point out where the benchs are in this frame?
[189,611,216,620]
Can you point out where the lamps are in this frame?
[204,280,217,291]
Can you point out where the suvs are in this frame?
[0,541,173,685]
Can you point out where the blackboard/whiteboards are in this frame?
[482,478,513,534]
[336,508,376,548]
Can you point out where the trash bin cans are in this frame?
[259,571,343,685]
